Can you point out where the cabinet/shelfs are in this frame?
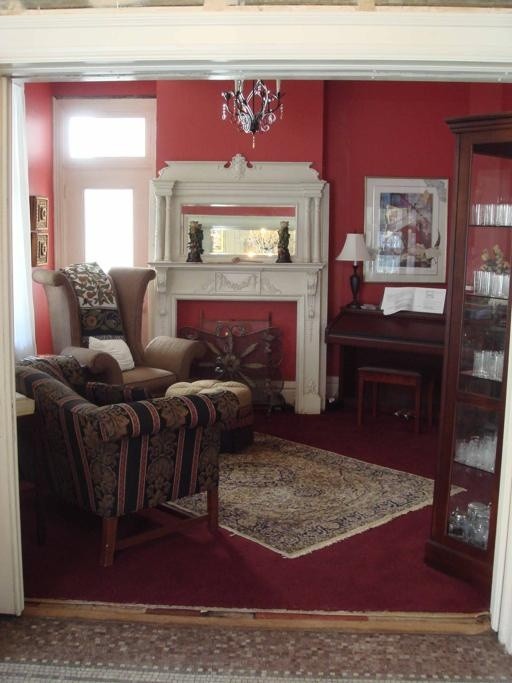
[425,110,511,586]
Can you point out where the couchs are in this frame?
[31,268,206,400]
[13,354,244,549]
[166,378,253,452]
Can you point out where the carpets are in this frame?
[156,431,469,557]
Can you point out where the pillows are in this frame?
[88,336,136,373]
[86,378,150,404]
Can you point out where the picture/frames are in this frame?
[362,175,451,288]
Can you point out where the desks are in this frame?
[326,308,447,399]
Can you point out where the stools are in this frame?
[357,360,436,434]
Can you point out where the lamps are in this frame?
[335,231,375,308]
[219,79,285,150]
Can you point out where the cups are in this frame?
[449,501,491,550]
[473,269,511,299]
[473,349,505,381]
[471,202,512,225]
[455,435,498,472]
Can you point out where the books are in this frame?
[381,287,447,316]
